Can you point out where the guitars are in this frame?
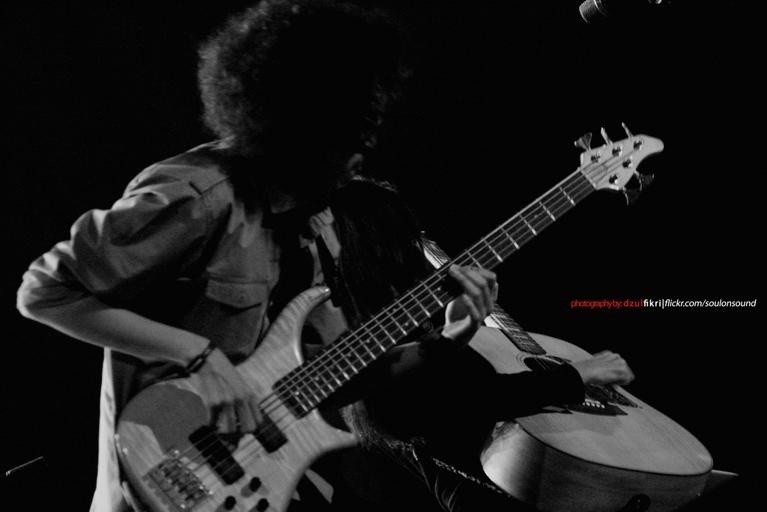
[418,236,713,511]
[114,124,664,511]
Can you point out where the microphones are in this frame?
[578,0,611,26]
[347,153,370,180]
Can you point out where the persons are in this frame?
[305,183,634,512]
[15,0,500,512]
